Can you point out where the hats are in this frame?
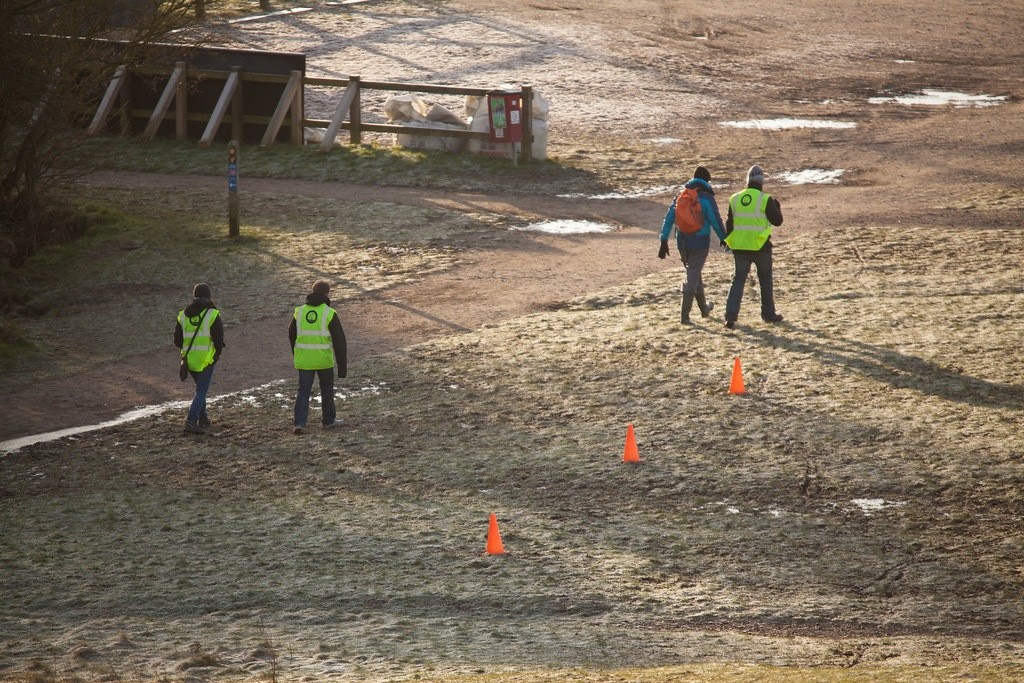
[694,166,711,180]
[748,165,764,183]
[193,283,211,298]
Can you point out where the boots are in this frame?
[680,291,695,325]
[696,294,714,318]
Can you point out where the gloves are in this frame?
[719,240,728,251]
[658,240,670,259]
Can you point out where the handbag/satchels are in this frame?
[180,357,188,380]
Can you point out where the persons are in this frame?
[173,283,225,434]
[657,167,725,324]
[723,164,784,326]
[288,280,347,434]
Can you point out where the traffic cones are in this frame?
[728,355,748,395]
[483,512,511,556]
[622,423,646,463]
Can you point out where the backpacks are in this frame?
[675,185,703,233]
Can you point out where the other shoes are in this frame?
[764,315,782,322]
[183,421,205,436]
[725,320,734,327]
[293,426,305,435]
[198,420,211,433]
[321,420,345,428]
